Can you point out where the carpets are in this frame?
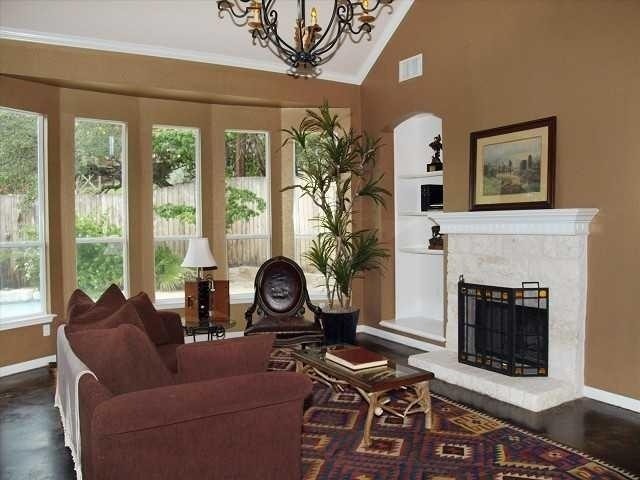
[267,340,640,480]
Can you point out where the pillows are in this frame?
[66,289,94,319]
[66,323,176,396]
[70,283,127,325]
[65,301,146,335]
[127,290,171,345]
[175,334,274,384]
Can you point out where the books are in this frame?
[325,347,389,371]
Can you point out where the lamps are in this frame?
[179,237,217,280]
[216,0,395,80]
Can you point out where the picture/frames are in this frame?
[469,116,557,211]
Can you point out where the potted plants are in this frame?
[275,95,392,343]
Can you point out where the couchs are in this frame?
[50,309,313,480]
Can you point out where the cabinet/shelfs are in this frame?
[377,112,445,342]
[407,207,599,413]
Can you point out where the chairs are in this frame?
[243,255,327,350]
